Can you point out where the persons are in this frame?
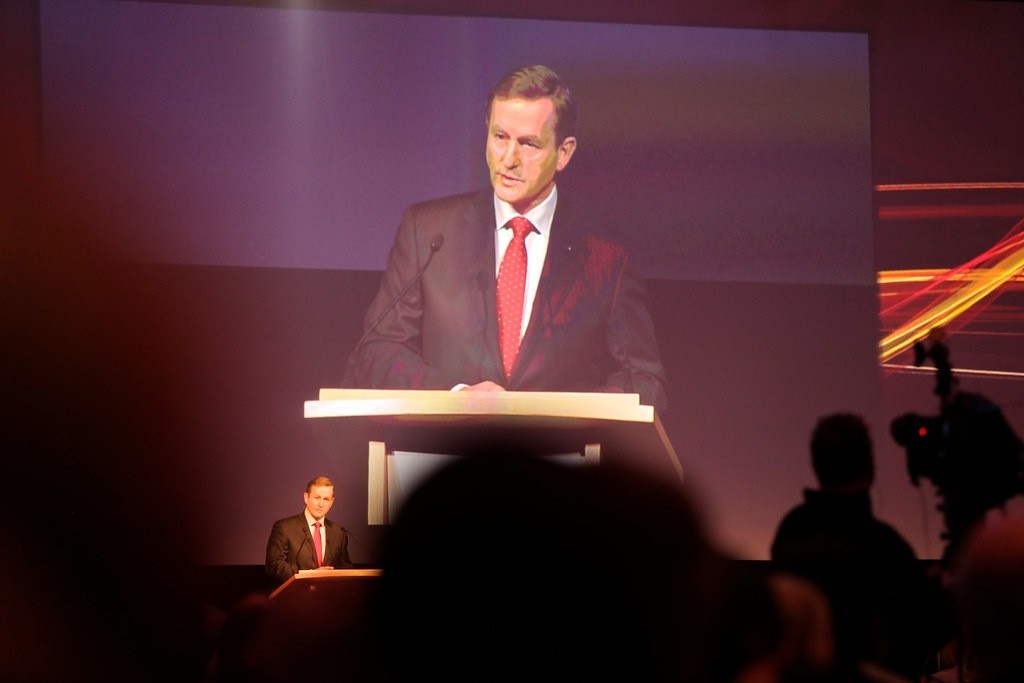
[362,63,668,416]
[334,389,1024,682]
[267,477,354,582]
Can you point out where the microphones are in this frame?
[341,525,383,569]
[340,233,444,388]
[296,528,307,571]
[559,243,636,394]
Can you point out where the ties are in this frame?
[312,521,323,568]
[495,216,536,382]
